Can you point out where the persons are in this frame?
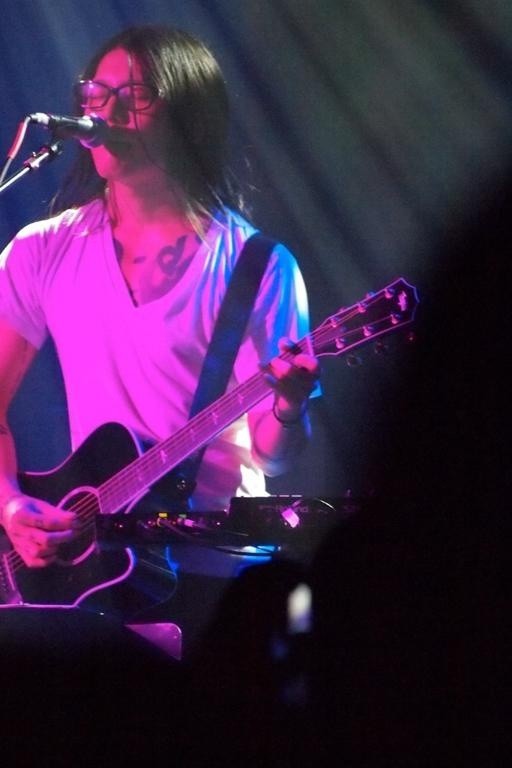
[1,21,322,665]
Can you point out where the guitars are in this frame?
[2,275,418,610]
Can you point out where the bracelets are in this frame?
[272,399,308,433]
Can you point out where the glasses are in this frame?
[72,79,159,112]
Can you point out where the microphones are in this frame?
[36,109,110,148]
